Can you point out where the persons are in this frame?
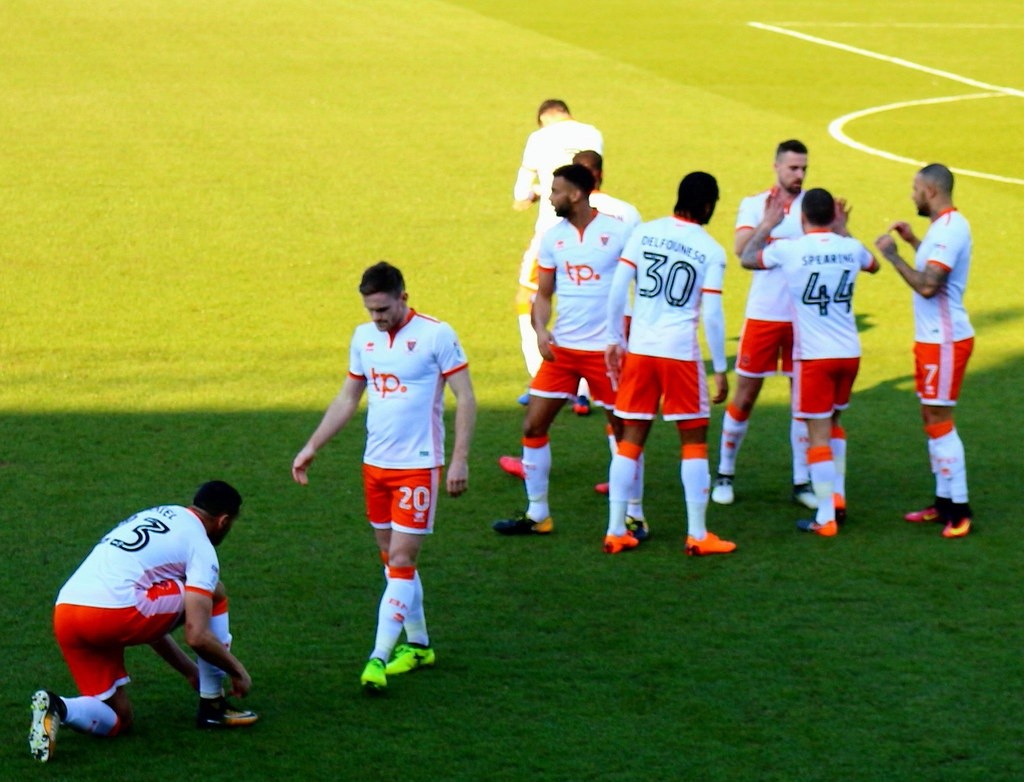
[872,161,980,540]
[288,260,480,694]
[495,146,647,496]
[739,185,881,541]
[488,159,658,541]
[599,166,745,560]
[511,94,602,422]
[709,135,824,512]
[25,475,266,763]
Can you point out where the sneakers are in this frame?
[685,532,736,556]
[836,509,846,526]
[710,476,735,506]
[594,481,610,493]
[196,696,257,724]
[602,532,640,553]
[623,518,650,538]
[382,643,435,679]
[794,490,820,511]
[28,689,65,767]
[571,396,591,416]
[360,658,391,693]
[496,455,526,481]
[938,517,974,537]
[796,518,839,535]
[492,511,554,533]
[903,504,953,523]
[517,388,532,405]
